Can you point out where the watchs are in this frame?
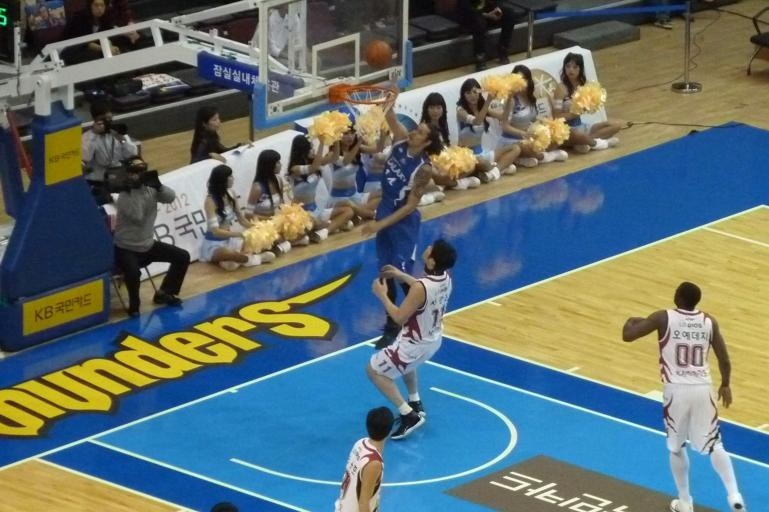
[119,138,125,144]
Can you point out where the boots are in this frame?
[416,137,618,206]
[219,210,354,271]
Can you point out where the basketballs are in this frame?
[367,40,393,69]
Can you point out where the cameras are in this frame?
[99,119,129,135]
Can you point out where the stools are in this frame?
[24,1,327,115]
[328,1,558,64]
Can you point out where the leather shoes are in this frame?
[154,291,182,306]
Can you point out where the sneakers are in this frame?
[670,499,693,512]
[391,399,426,439]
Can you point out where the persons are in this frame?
[114,156,190,318]
[362,86,442,348]
[23,1,145,100]
[247,148,323,257]
[249,3,321,74]
[333,407,393,512]
[190,106,254,164]
[551,52,621,154]
[285,91,480,245]
[623,281,746,511]
[456,0,516,72]
[499,66,568,166]
[327,1,400,36]
[456,77,521,183]
[198,164,275,271]
[211,500,239,512]
[366,240,457,439]
[81,99,138,206]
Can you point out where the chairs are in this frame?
[745,6,769,75]
[96,206,158,313]
